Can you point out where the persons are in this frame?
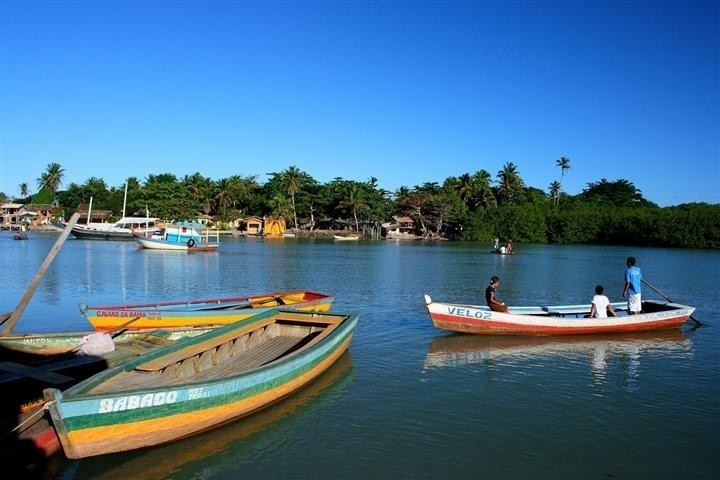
[494,236,513,254]
[623,256,643,314]
[486,277,509,312]
[588,285,617,318]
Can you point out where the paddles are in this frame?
[642,279,703,325]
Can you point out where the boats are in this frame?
[334,234,359,240]
[42,308,362,461]
[490,249,515,255]
[78,290,336,332]
[424,294,696,336]
[62,216,162,241]
[13,231,27,241]
[133,223,220,252]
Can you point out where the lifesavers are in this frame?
[187,240,194,247]
